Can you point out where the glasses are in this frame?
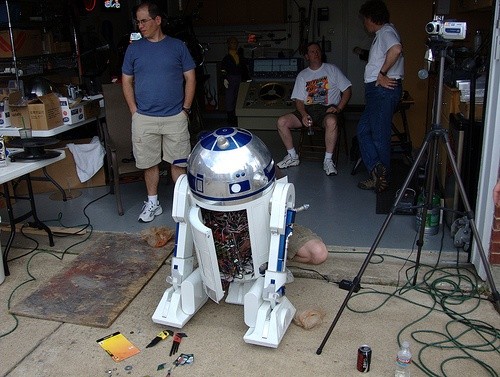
[135,19,153,25]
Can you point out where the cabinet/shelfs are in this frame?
[426,75,461,190]
[0,22,78,77]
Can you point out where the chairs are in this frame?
[102,83,171,216]
[298,110,347,169]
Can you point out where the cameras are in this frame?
[426,15,468,40]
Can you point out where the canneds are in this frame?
[307,120,314,135]
[356,345,372,372]
[0,138,6,161]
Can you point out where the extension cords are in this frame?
[339,279,361,292]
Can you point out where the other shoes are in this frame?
[360,170,377,189]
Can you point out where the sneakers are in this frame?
[323,158,338,175]
[138,201,163,221]
[277,154,300,168]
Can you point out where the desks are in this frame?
[0,115,103,138]
[0,148,66,276]
[391,96,416,152]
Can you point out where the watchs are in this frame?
[380,71,387,76]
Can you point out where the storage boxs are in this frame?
[0,28,71,58]
[0,93,104,131]
[0,138,106,208]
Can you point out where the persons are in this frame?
[221,37,244,125]
[276,41,353,176]
[357,1,405,193]
[287,223,328,265]
[122,3,196,223]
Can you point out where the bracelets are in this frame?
[183,107,190,111]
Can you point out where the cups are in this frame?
[19,128,32,140]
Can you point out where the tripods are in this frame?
[317,45,500,353]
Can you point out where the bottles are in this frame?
[415,190,441,236]
[307,116,315,135]
[394,341,413,377]
